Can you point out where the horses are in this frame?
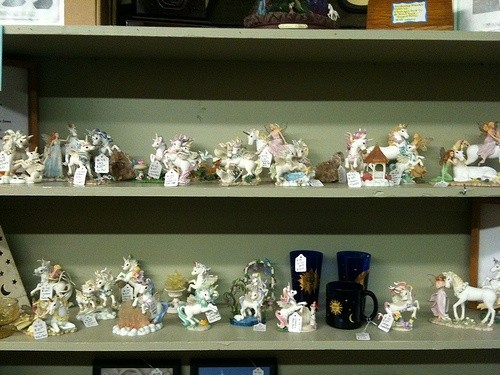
[444,271,497,328]
[327,3,340,21]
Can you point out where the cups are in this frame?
[326,281,378,330]
[336,251,371,315]
[289,250,323,311]
[0,297,19,325]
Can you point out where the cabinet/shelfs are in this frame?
[0,26,500,375]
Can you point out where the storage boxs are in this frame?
[366,0,455,29]
[64,0,118,26]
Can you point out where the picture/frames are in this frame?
[0,60,38,150]
[471,197,500,310]
[93,359,278,375]
